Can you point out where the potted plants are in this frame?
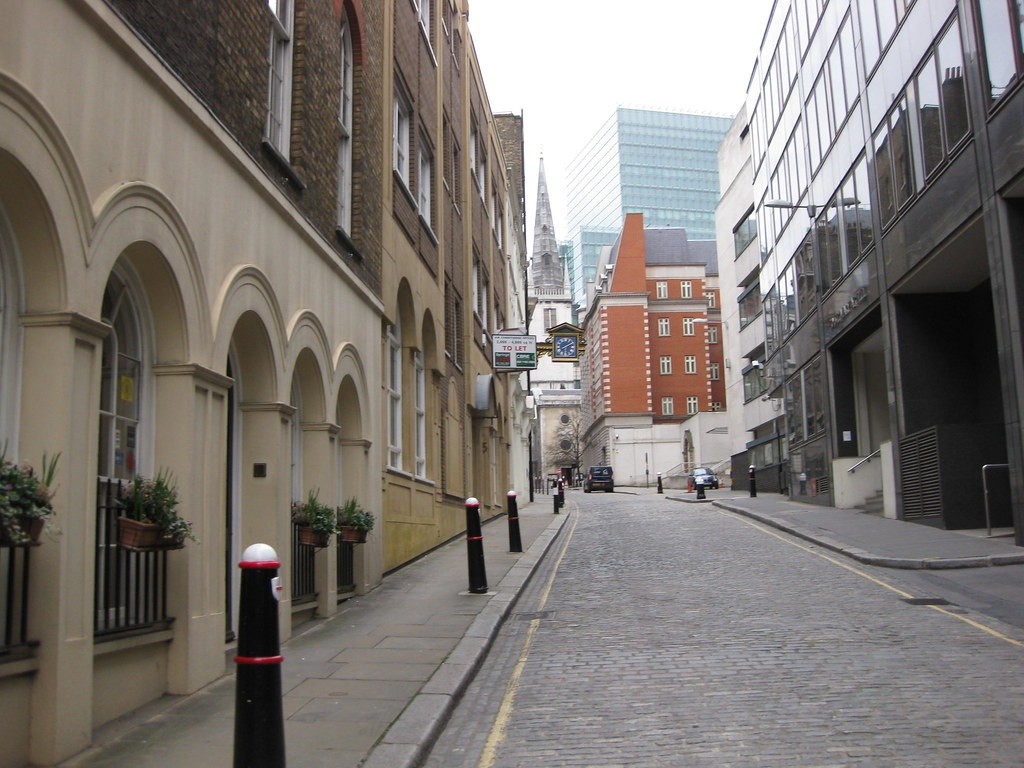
[292,486,342,547]
[0,437,67,548]
[115,463,203,552]
[337,495,376,542]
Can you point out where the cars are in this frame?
[688,466,719,490]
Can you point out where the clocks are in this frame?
[554,336,577,358]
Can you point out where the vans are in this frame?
[583,466,614,493]
